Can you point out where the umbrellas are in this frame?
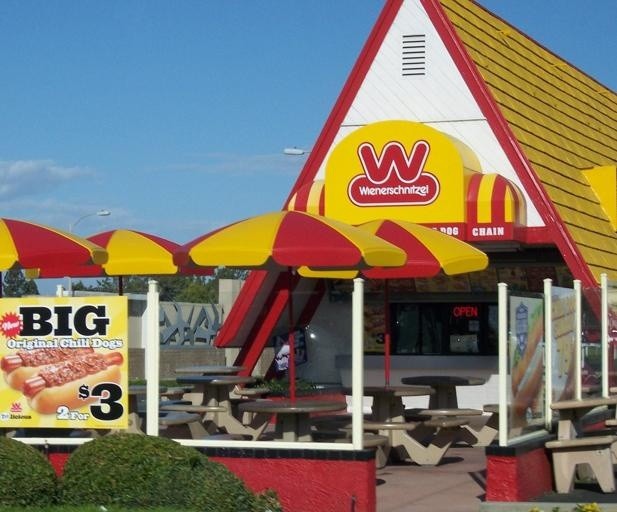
[173,210,408,405]
[1,217,107,296]
[26,227,214,295]
[292,217,490,385]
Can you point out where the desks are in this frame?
[401,375,487,409]
[549,400,617,439]
[176,375,258,440]
[174,367,248,373]
[128,385,167,412]
[238,400,348,443]
[341,385,434,467]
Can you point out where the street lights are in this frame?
[68,209,112,296]
[283,148,312,155]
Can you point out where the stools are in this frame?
[418,408,484,415]
[161,413,200,440]
[479,404,502,447]
[363,420,415,469]
[364,434,388,448]
[160,404,227,439]
[235,387,271,425]
[424,418,469,465]
[545,436,616,495]
[161,386,187,401]
